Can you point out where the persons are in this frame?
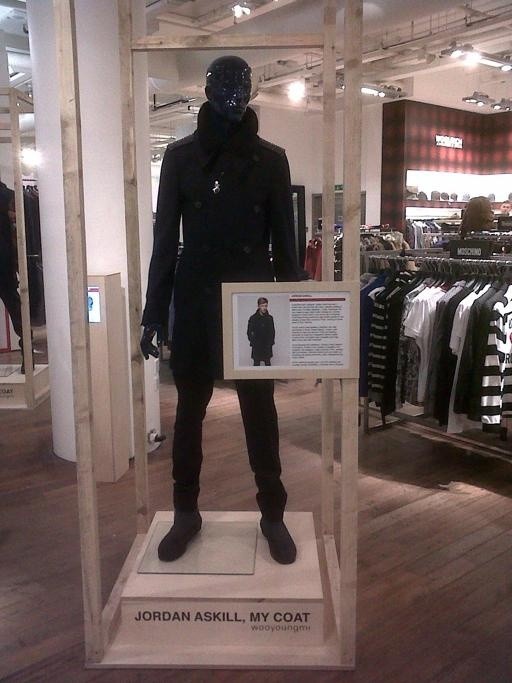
[140,56,315,565]
[247,297,275,366]
[500,200,512,213]
[0,181,35,374]
[458,196,495,234]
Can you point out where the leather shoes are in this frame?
[261,518,296,564]
[158,510,202,561]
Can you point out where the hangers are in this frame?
[345,228,511,302]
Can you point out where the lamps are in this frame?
[228,0,253,21]
[439,39,512,74]
[459,86,512,113]
